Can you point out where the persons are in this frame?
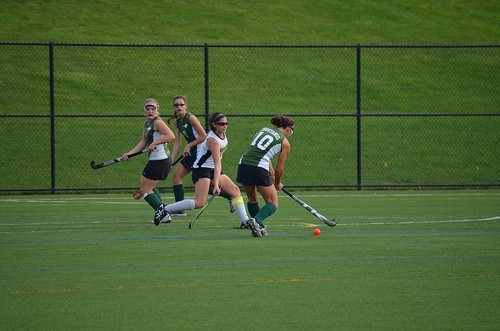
[236,115,294,237]
[154,112,251,228]
[122,98,175,223]
[167,96,207,216]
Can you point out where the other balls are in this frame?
[314,228,320,235]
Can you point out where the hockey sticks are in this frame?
[189,185,224,229]
[133,155,184,200]
[281,187,337,227]
[90,149,149,169]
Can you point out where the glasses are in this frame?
[216,121,228,126]
[144,105,156,111]
[289,128,293,136]
[174,103,185,107]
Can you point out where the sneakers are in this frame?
[154,203,168,226]
[151,213,172,223]
[170,211,187,217]
[240,222,262,229]
[246,218,262,237]
[228,198,235,213]
[260,228,266,236]
